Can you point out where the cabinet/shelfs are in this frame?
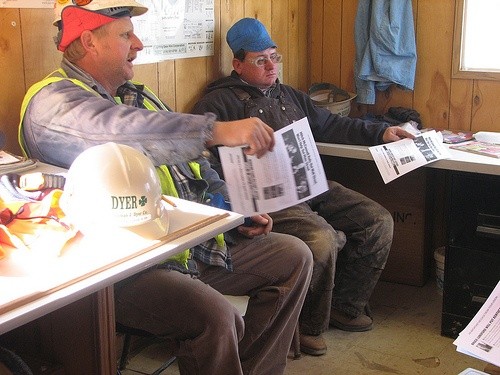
[440,171,500,342]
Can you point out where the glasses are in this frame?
[243,53,285,66]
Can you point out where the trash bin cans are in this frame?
[433,247,446,297]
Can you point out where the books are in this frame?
[449,140,500,159]
[420,127,475,143]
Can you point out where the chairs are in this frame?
[116,293,250,375]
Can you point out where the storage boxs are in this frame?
[328,156,433,287]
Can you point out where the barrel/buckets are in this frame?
[433,246,445,288]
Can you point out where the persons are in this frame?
[194,18,416,356]
[18,0,312,375]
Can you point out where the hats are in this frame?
[226,17,277,54]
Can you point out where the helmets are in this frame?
[307,82,357,118]
[59,143,171,245]
[50,0,148,51]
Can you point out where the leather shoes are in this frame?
[301,332,327,357]
[331,308,374,332]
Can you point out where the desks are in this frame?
[0,163,246,375]
[313,139,500,175]
